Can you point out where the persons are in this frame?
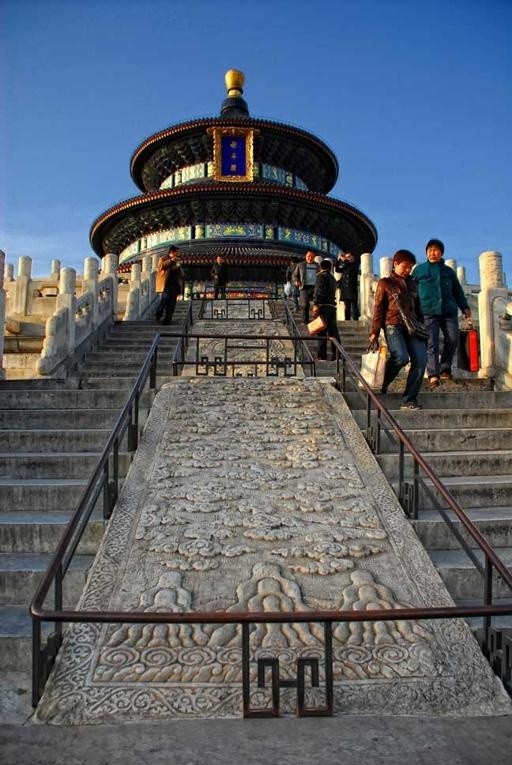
[209,252,230,298]
[284,237,471,412]
[154,243,188,327]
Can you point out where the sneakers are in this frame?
[400,404,423,411]
[430,377,438,383]
[440,372,453,380]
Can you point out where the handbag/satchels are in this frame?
[404,317,430,341]
[457,329,479,372]
[307,316,326,336]
[358,353,386,390]
